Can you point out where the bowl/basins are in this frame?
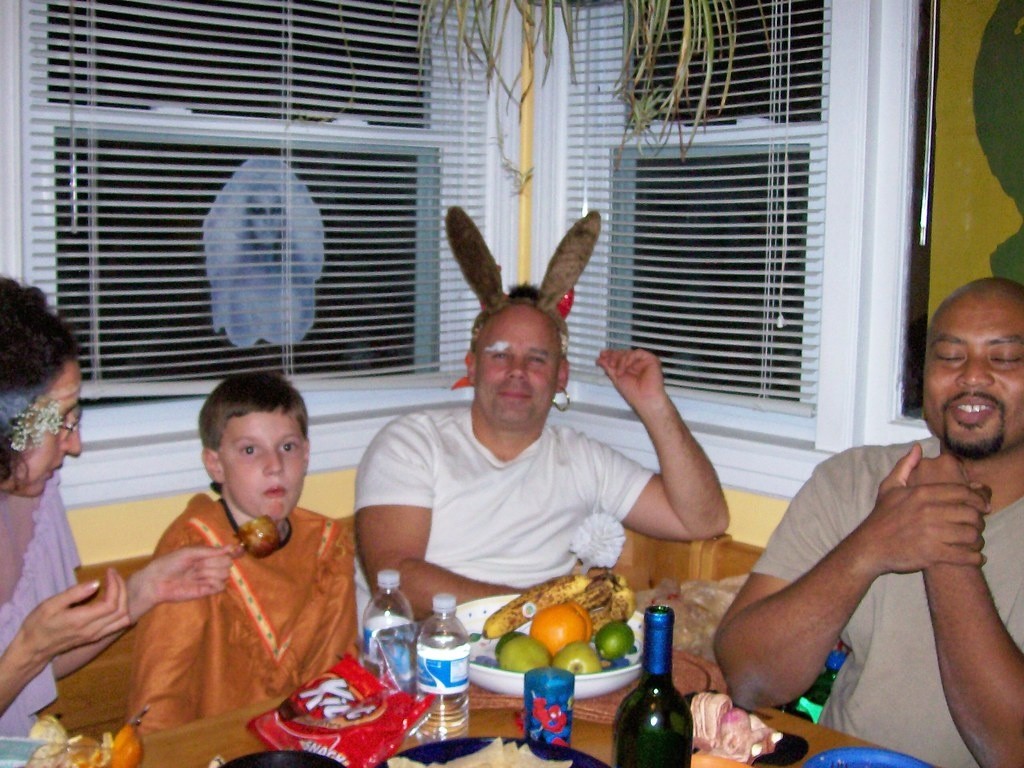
[448,595,658,697]
[0,735,112,768]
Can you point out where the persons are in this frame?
[717,275,1024,768]
[348,283,731,637]
[0,277,237,743]
[115,370,357,737]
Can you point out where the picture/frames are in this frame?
[900,0,1024,421]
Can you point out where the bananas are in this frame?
[482,571,635,632]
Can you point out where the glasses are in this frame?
[27,406,81,442]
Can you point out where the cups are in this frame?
[523,669,573,745]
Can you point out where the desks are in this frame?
[141,690,879,768]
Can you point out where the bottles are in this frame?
[795,651,843,725]
[416,588,469,739]
[611,605,694,768]
[361,570,413,680]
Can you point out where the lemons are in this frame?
[495,631,527,660]
[596,622,635,659]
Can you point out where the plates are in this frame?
[216,750,346,768]
[374,737,610,768]
[803,747,933,768]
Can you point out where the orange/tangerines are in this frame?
[529,602,591,655]
[25,714,143,768]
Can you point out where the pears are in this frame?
[499,636,601,675]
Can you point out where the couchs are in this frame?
[41,508,771,736]
